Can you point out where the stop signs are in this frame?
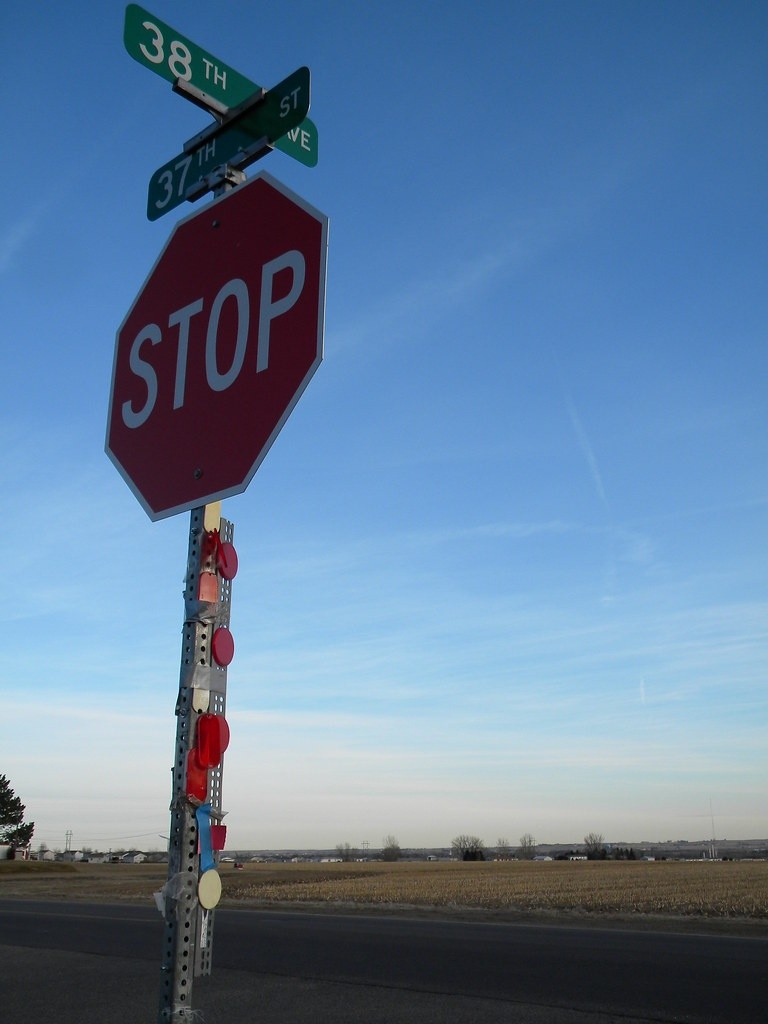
[105,169,331,523]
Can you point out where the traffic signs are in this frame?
[147,66,309,223]
[124,3,320,168]
